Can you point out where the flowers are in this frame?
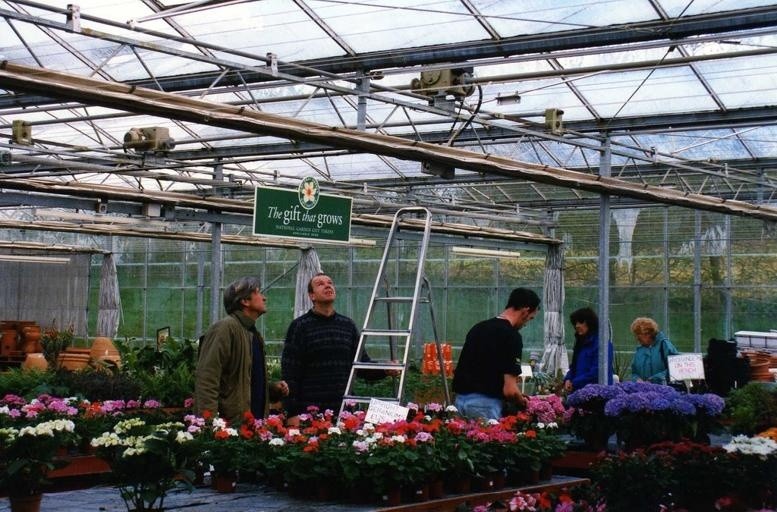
[0,380,776,498]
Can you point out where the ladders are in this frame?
[336,206,451,427]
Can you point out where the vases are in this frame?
[10,441,606,511]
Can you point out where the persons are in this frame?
[629,316,680,386]
[282,272,403,412]
[452,288,540,422]
[194,275,290,423]
[564,308,614,395]
